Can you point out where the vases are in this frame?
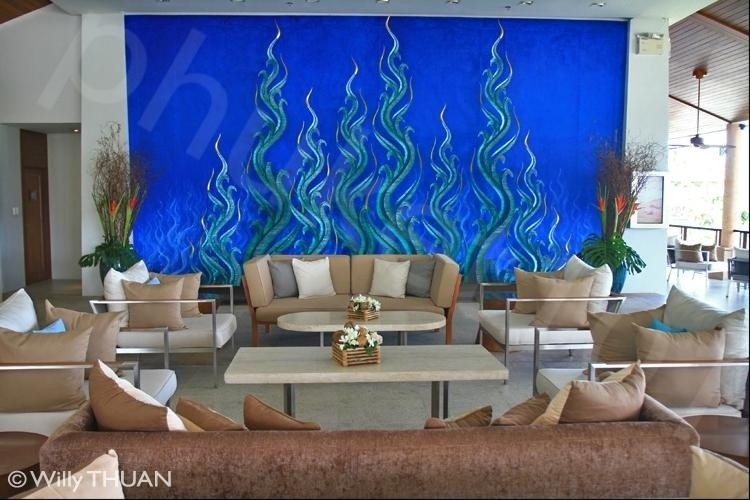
[346,308,378,321]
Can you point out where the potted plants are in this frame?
[74,126,149,283]
[568,131,669,310]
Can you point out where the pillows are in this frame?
[368,257,410,299]
[664,284,749,411]
[564,254,613,312]
[535,364,648,424]
[687,443,749,499]
[630,320,727,407]
[528,275,597,326]
[0,287,129,414]
[266,257,307,299]
[103,259,202,331]
[405,257,437,298]
[291,256,336,299]
[243,392,322,432]
[512,266,565,314]
[584,305,667,374]
[493,390,547,428]
[89,360,186,433]
[423,402,493,429]
[8,449,125,498]
[175,395,248,433]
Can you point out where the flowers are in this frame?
[328,321,384,355]
[344,292,382,312]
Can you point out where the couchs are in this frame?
[241,253,463,346]
[41,391,706,500]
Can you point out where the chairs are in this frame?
[88,285,238,388]
[0,327,179,437]
[667,234,749,299]
[531,284,750,418]
[477,282,627,386]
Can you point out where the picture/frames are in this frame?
[629,169,665,229]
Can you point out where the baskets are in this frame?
[347,308,381,323]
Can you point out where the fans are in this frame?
[669,65,737,153]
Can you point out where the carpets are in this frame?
[138,359,543,429]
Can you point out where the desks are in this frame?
[0,431,48,493]
[684,415,749,462]
